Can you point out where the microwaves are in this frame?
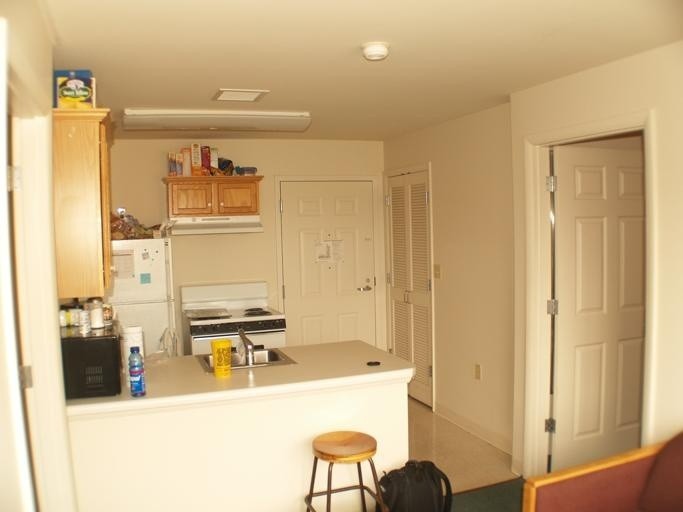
[59,319,125,399]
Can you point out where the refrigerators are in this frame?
[102,236,177,358]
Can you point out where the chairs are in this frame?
[521,431,683,512]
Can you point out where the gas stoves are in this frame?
[182,301,287,338]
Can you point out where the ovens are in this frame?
[181,328,286,355]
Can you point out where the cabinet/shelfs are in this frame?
[387,170,432,408]
[52,107,112,298]
[160,176,261,218]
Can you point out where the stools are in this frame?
[302,430,390,511]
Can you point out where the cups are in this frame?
[209,338,230,379]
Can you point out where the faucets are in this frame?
[239,329,255,367]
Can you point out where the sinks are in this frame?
[195,348,298,374]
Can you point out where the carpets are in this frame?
[443,475,526,512]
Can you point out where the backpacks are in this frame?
[375,460,452,512]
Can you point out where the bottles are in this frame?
[126,347,145,397]
[58,295,114,337]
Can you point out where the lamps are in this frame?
[359,40,391,60]
